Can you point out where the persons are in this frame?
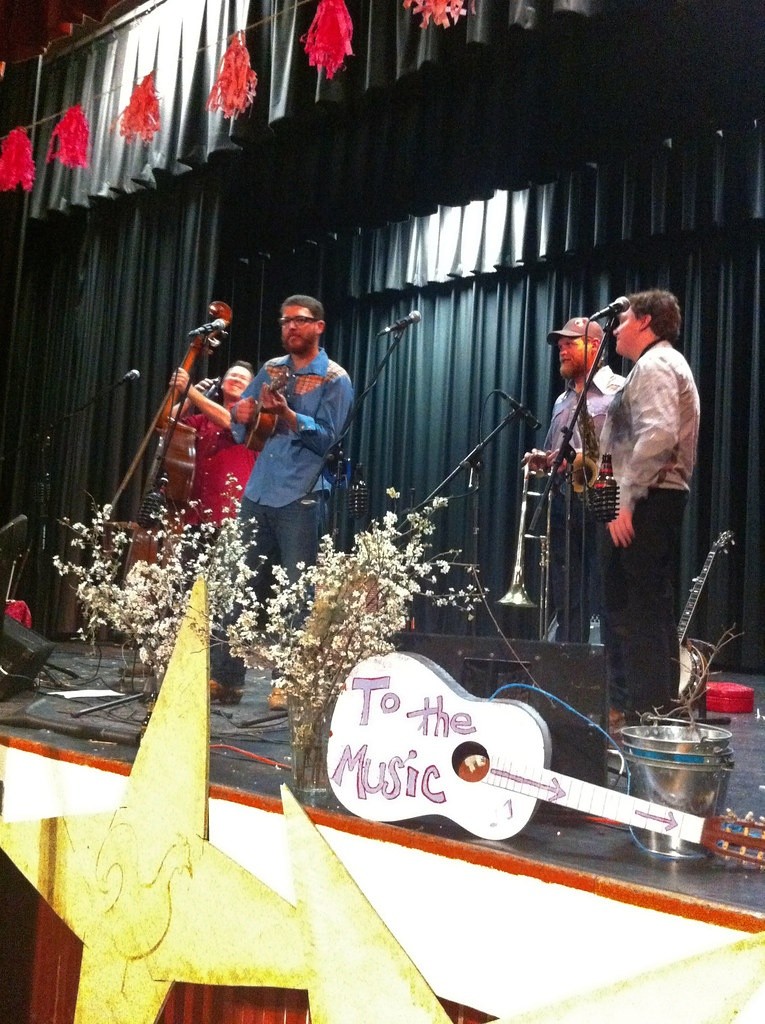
[209,294,355,711]
[544,289,700,756]
[155,359,259,705]
[522,315,625,647]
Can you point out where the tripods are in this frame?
[72,336,208,720]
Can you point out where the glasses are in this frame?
[279,316,317,325]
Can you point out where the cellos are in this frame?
[124,299,233,574]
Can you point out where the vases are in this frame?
[139,665,168,704]
[286,695,336,792]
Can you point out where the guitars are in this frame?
[326,651,765,870]
[244,370,294,452]
[675,530,737,716]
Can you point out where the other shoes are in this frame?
[120,664,154,677]
[608,709,625,726]
[610,726,626,740]
[267,688,288,710]
[209,680,242,705]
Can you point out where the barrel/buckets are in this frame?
[620,712,735,858]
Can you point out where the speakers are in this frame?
[0,613,57,703]
[392,631,609,814]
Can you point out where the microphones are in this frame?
[188,319,226,336]
[499,390,542,432]
[590,296,630,322]
[124,369,140,381]
[379,311,421,336]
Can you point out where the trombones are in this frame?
[492,449,555,641]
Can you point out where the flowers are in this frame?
[54,472,267,675]
[180,488,491,745]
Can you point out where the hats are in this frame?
[545,317,604,347]
